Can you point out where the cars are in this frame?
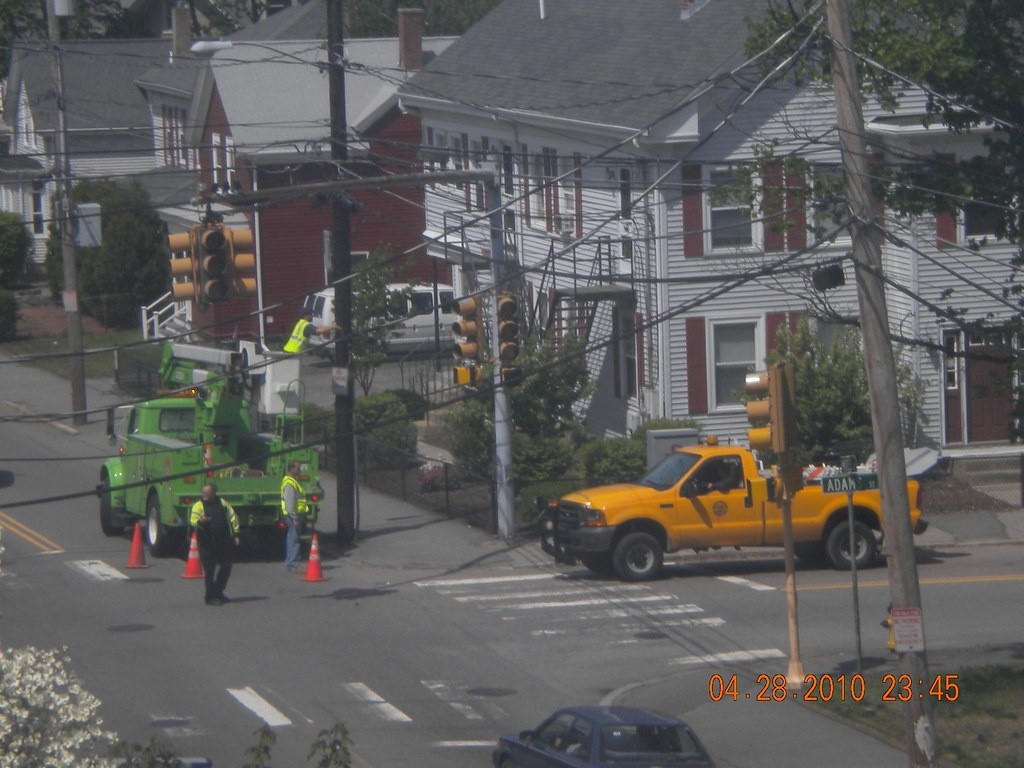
[490,702,717,768]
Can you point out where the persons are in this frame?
[283,301,343,355]
[190,483,241,605]
[702,466,737,493]
[281,459,311,573]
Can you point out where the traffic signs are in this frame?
[823,474,877,494]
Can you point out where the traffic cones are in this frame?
[120,520,149,570]
[178,531,207,580]
[298,535,332,584]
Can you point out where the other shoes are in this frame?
[205,594,229,605]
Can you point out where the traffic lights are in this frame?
[746,465,781,502]
[452,364,483,387]
[196,225,230,304]
[498,294,521,362]
[226,228,257,300]
[744,360,799,456]
[451,294,482,363]
[165,225,207,307]
[500,366,522,386]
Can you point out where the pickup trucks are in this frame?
[538,436,931,582]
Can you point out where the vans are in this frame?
[302,282,463,364]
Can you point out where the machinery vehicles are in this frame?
[95,328,325,562]
